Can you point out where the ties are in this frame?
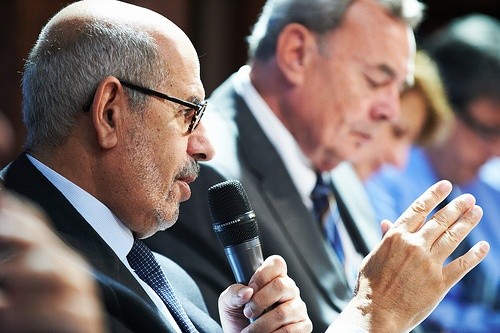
[311,169,345,264]
[125,234,198,333]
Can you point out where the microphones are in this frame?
[206,179,273,325]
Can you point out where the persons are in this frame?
[365,14,499,333]
[0,0,490,332]
[142,0,421,332]
[347,47,456,184]
[1,191,102,333]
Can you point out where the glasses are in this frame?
[456,103,500,144]
[82,79,207,134]
